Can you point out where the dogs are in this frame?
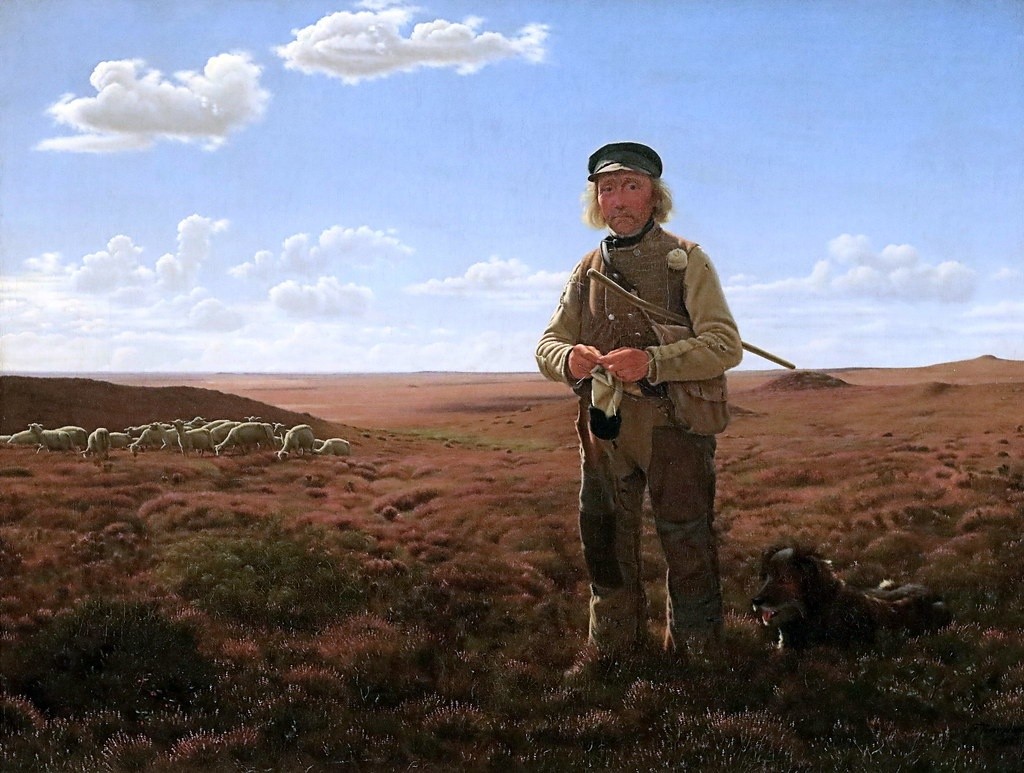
[749,545,957,659]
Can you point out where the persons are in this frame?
[533,142,743,682]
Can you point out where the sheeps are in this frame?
[1,415,350,462]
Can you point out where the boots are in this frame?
[664,569,724,675]
[564,582,647,689]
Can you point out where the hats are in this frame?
[587,142,663,182]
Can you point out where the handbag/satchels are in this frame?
[650,323,730,436]
